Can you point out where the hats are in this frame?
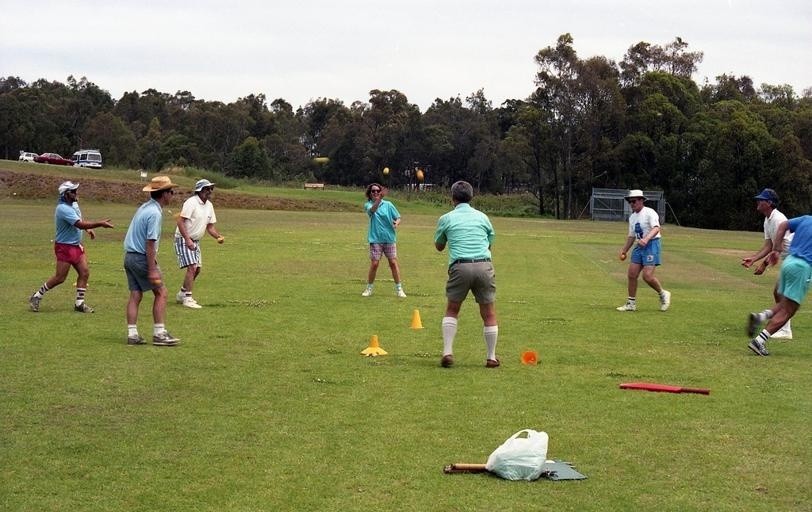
[624,190,646,202]
[142,176,178,191]
[58,181,80,194]
[194,179,215,191]
[755,188,776,205]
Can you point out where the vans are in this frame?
[71,150,102,168]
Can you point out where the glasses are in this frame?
[205,188,213,191]
[628,200,636,203]
[372,190,380,193]
[166,191,173,195]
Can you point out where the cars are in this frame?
[34,152,74,166]
[19,152,39,163]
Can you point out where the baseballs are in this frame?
[621,254,625,259]
[417,170,424,180]
[314,157,329,163]
[155,280,162,286]
[383,167,389,174]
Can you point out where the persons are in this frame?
[174,179,225,309]
[748,215,812,358]
[616,189,672,312]
[361,183,407,298]
[741,188,793,339]
[434,181,501,368]
[123,176,181,346]
[30,181,114,312]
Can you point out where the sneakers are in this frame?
[75,302,95,313]
[28,293,42,311]
[748,313,792,356]
[362,288,372,296]
[128,337,146,344]
[397,290,407,297]
[442,355,453,366]
[153,330,179,345]
[176,293,203,309]
[659,291,671,311]
[487,359,500,367]
[616,304,636,311]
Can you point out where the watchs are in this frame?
[764,262,769,268]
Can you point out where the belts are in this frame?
[452,259,492,263]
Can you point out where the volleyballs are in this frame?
[218,236,224,242]
